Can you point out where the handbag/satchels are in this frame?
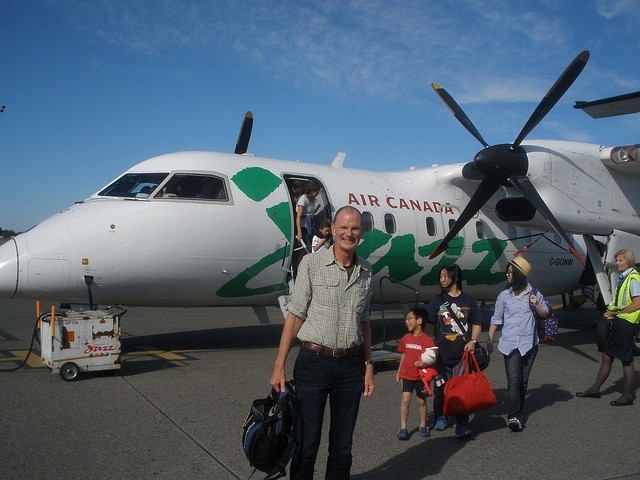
[470,341,490,371]
[533,302,558,342]
[442,346,496,415]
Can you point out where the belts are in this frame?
[302,339,363,357]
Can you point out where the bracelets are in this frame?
[365,359,374,366]
[470,338,477,343]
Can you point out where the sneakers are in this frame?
[419,427,430,438]
[508,417,523,432]
[455,425,471,438]
[398,429,409,440]
[434,416,448,431]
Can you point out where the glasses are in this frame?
[506,270,512,277]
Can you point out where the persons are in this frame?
[270,206,377,478]
[312,219,330,253]
[487,255,549,432]
[576,248,640,406]
[396,306,438,439]
[293,184,322,240]
[426,264,484,437]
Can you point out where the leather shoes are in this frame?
[575,392,601,398]
[610,398,634,406]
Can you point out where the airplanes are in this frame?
[0,51,640,305]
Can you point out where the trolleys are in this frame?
[371,276,419,375]
[40,304,136,381]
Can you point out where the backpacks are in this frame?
[241,379,302,480]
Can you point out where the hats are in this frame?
[508,256,531,277]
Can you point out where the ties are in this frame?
[617,274,624,288]
